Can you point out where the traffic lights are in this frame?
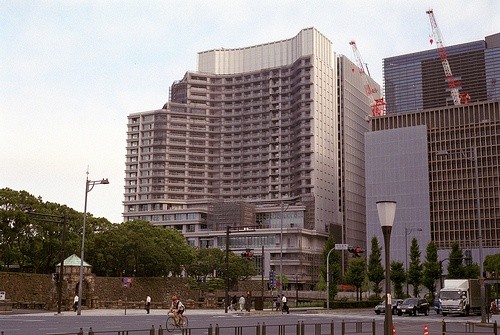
[347,247,365,253]
[241,252,253,257]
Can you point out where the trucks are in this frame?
[438,278,488,317]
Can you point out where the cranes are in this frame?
[349,41,388,117]
[425,8,472,105]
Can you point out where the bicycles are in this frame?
[166,309,188,333]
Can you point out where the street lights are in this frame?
[404,227,423,298]
[77,164,109,313]
[437,138,484,282]
[279,200,303,300]
[375,200,397,335]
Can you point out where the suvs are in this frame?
[374,298,403,315]
[396,297,430,316]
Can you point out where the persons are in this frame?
[231,295,237,311]
[169,296,185,326]
[282,294,289,314]
[74,294,78,312]
[145,295,151,314]
[239,295,245,311]
[276,295,281,311]
[490,300,496,315]
